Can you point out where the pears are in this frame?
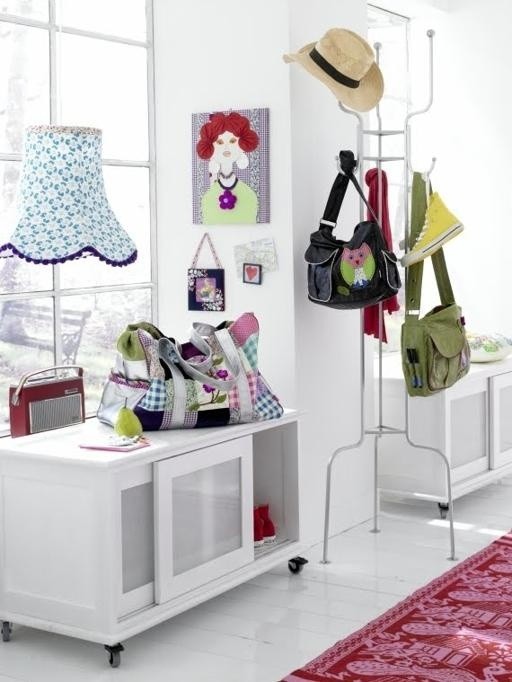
[115,396,144,438]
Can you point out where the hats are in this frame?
[282,25,386,113]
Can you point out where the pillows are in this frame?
[462,332,512,362]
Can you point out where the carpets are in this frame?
[275,526,511,682]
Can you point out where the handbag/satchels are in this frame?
[96,309,286,432]
[399,166,472,397]
[186,233,226,311]
[303,151,400,310]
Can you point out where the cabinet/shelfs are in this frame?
[1,402,313,669]
[375,346,511,522]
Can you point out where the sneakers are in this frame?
[253,505,277,548]
[400,193,464,268]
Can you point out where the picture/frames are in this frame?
[187,266,226,313]
[242,263,262,286]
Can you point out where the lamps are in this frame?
[0,121,139,270]
